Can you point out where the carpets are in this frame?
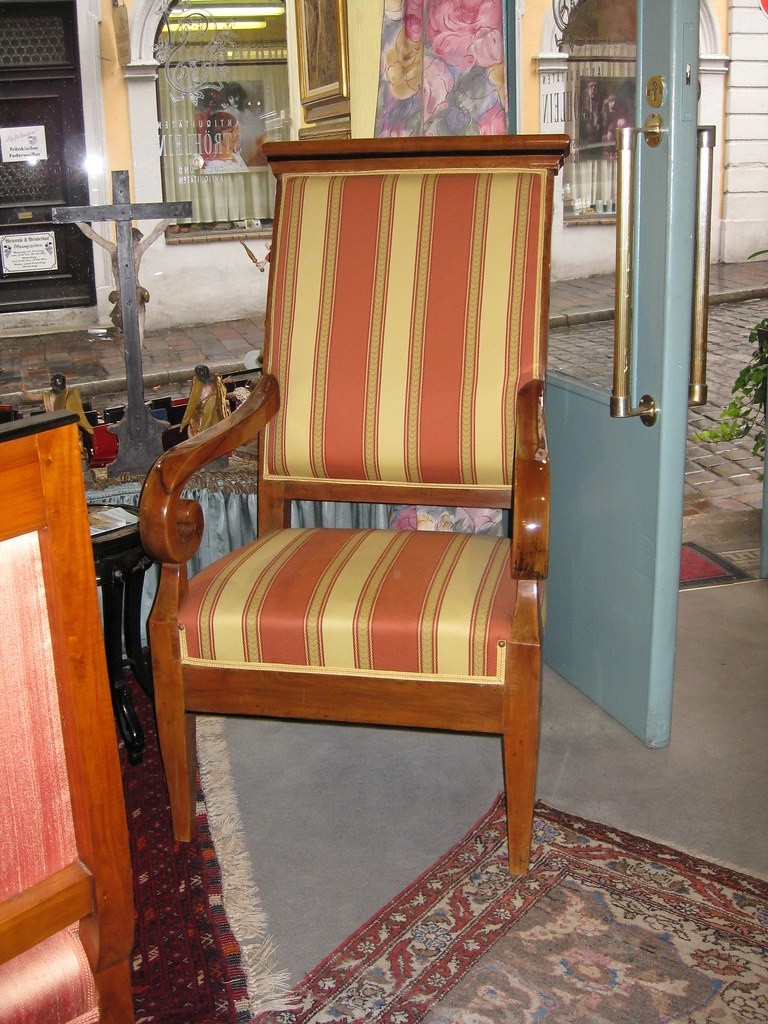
[111,668,305,1024]
[247,792,768,1024]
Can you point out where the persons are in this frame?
[77,218,172,351]
[179,365,231,439]
[18,374,94,447]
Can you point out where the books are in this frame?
[89,506,142,542]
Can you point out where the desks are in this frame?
[87,502,154,766]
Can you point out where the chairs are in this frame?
[0,412,136,1024]
[141,136,572,877]
